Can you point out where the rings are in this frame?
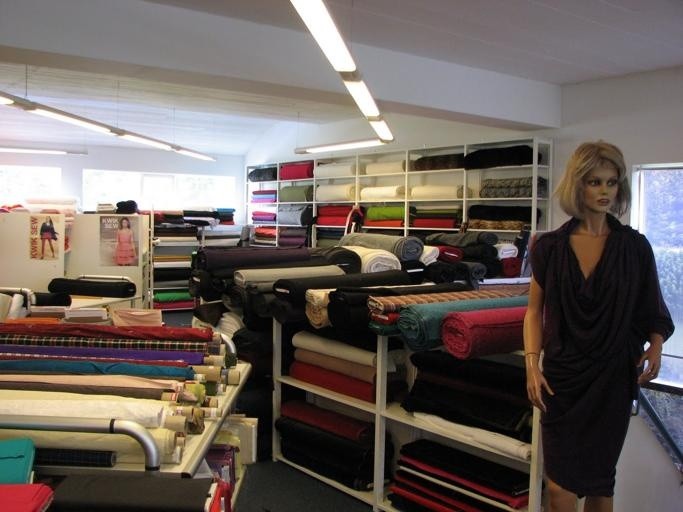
[650,374,655,379]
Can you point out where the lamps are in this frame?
[2,63,220,167]
[291,0,401,144]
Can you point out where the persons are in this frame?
[521,140,675,512]
[112,217,136,266]
[39,216,59,259]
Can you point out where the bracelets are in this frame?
[525,352,536,355]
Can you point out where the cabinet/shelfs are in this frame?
[246,138,553,512]
[1,209,246,512]
